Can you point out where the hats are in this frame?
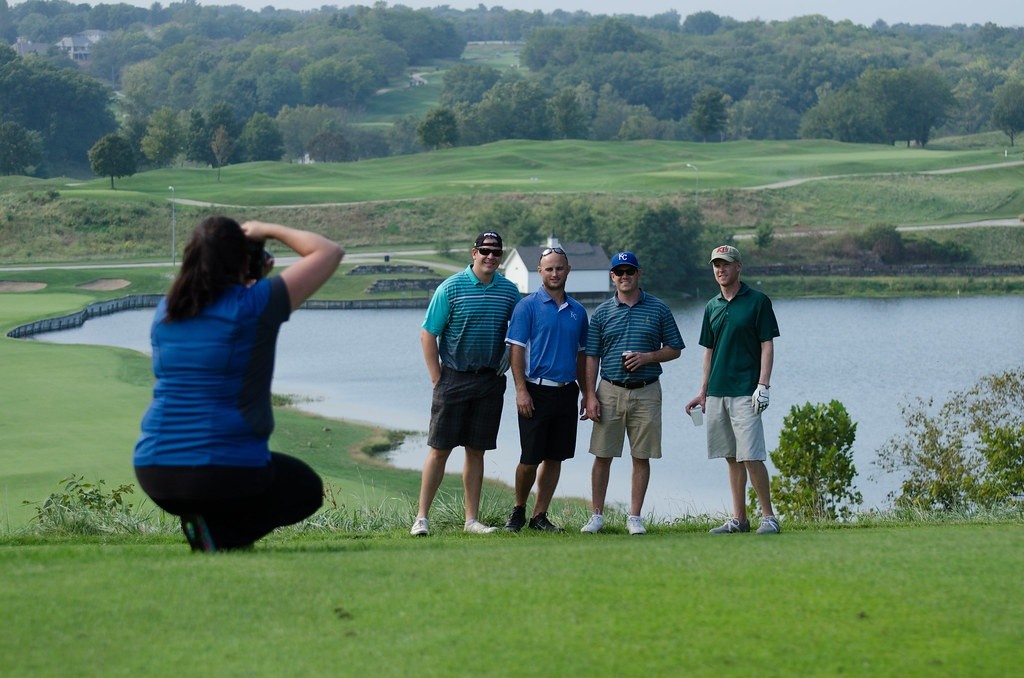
[611,252,639,270]
[472,231,502,249]
[709,245,742,265]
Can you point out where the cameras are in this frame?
[243,239,274,281]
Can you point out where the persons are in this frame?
[685,245,780,534]
[581,251,686,535]
[133,216,345,553]
[504,247,589,534]
[409,230,523,536]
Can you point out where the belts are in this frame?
[527,376,570,387]
[602,375,658,389]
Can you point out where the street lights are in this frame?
[686,163,699,205]
[168,184,175,268]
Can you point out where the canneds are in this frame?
[622,351,634,374]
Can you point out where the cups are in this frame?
[689,406,703,426]
[621,351,635,374]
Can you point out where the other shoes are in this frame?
[180,516,220,552]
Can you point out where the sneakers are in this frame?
[625,515,646,535]
[709,517,751,533]
[410,518,429,536]
[755,515,780,534]
[580,508,604,535]
[529,512,568,534]
[502,502,527,534]
[464,519,499,534]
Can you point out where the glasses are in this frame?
[613,268,638,277]
[475,248,503,257]
[540,248,568,262]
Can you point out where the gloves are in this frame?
[751,383,772,415]
